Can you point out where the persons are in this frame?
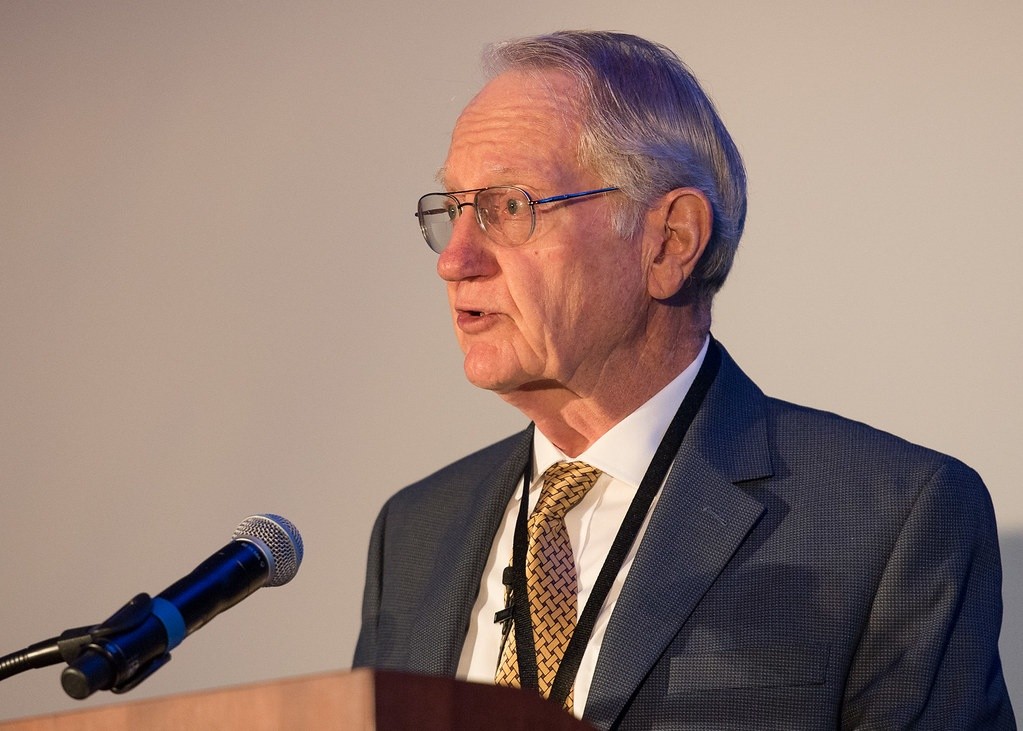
[351,24,1017,730]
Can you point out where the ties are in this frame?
[492,459,603,716]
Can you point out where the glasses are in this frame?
[415,185,620,255]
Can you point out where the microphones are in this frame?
[63,512,304,700]
